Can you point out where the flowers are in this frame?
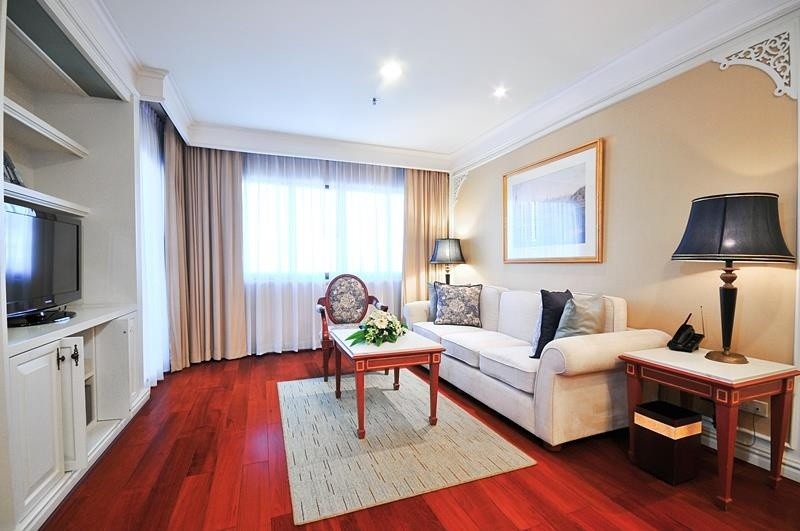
[345,309,409,347]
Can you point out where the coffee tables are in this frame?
[331,328,446,439]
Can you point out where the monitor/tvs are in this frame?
[5,202,82,327]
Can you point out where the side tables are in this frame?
[618,347,800,511]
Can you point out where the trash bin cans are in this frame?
[634,401,703,485]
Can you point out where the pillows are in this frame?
[428,282,471,322]
[434,281,483,328]
[554,295,605,339]
[529,289,574,358]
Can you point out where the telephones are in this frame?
[667,305,705,353]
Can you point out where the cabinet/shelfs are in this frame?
[0,0,140,531]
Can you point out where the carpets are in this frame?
[276,367,538,526]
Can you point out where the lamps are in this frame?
[671,192,796,365]
[430,239,466,284]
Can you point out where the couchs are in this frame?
[403,285,672,452]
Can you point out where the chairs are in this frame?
[317,274,388,382]
